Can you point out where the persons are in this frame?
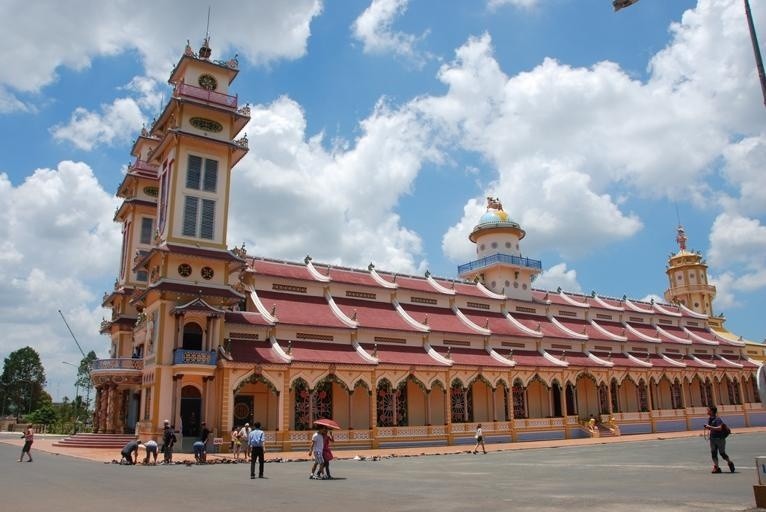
[473,424,488,455]
[230,423,252,459]
[120,440,141,465]
[192,441,205,465]
[200,421,209,461]
[189,412,198,437]
[248,422,266,479]
[704,405,735,473]
[317,426,335,480]
[308,426,330,480]
[586,414,601,430]
[17,424,33,462]
[163,420,174,463]
[144,440,159,465]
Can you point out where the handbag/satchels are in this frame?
[719,420,731,439]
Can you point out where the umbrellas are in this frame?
[313,419,340,436]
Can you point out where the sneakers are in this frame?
[310,474,334,480]
[712,465,721,473]
[728,462,734,472]
